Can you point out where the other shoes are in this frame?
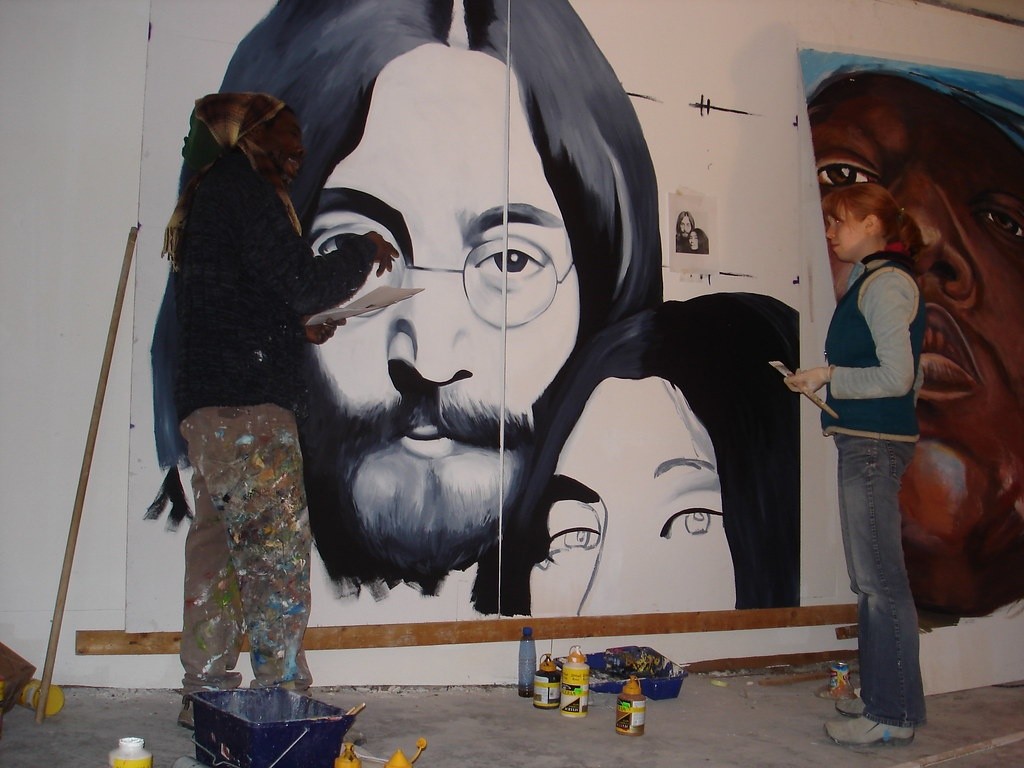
[823,716,914,748]
[176,699,195,729]
[834,698,867,717]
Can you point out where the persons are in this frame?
[785,186,925,748]
[676,210,708,253]
[173,93,400,728]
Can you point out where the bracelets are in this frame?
[828,365,835,378]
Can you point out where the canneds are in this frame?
[828,663,849,693]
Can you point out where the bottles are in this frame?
[533,654,561,710]
[335,743,362,768]
[108,737,152,768]
[561,645,590,718]
[518,627,536,698]
[615,675,646,736]
[384,737,427,768]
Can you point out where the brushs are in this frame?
[767,360,840,420]
